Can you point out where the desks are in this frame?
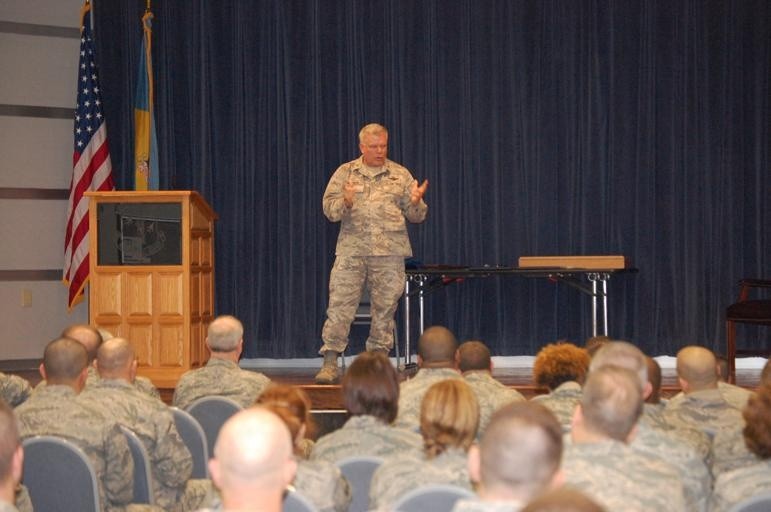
[395,266,638,371]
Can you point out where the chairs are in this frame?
[391,484,472,510]
[732,495,770,511]
[335,457,383,511]
[22,434,102,511]
[120,425,155,506]
[339,277,402,373]
[187,396,244,462]
[168,405,209,482]
[723,278,770,384]
[214,488,313,511]
[414,426,716,447]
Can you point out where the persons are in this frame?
[172,316,272,408]
[313,122,430,388]
[0,324,210,512]
[208,385,351,512]
[532,336,770,512]
[311,326,604,512]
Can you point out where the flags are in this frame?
[63,0,118,309]
[132,11,163,192]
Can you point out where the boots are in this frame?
[316,350,339,384]
